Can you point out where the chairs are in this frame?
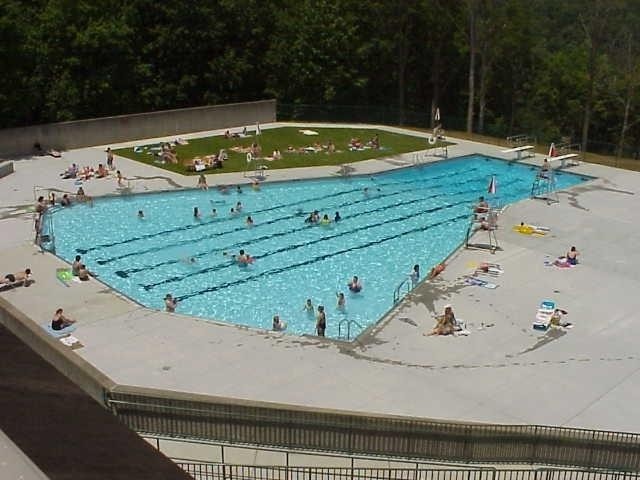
[61,127,390,181]
[1,272,34,291]
[533,299,557,329]
